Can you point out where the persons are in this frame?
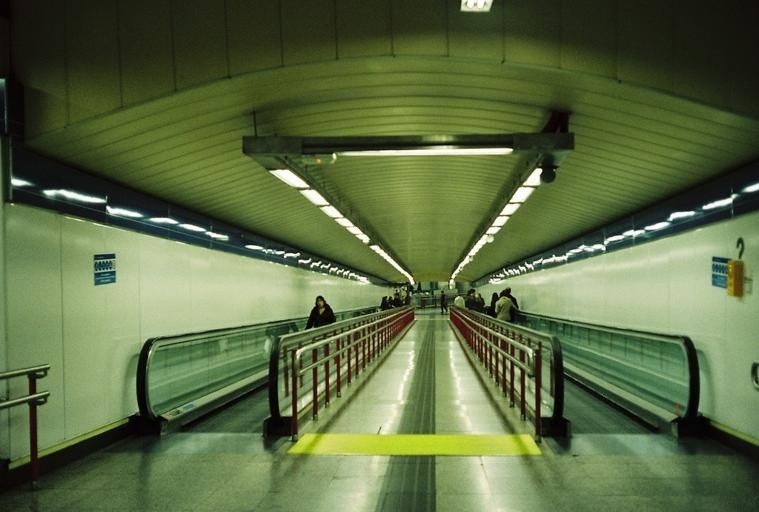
[304,295,336,330]
[380,285,519,322]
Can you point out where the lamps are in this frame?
[460,0,493,14]
